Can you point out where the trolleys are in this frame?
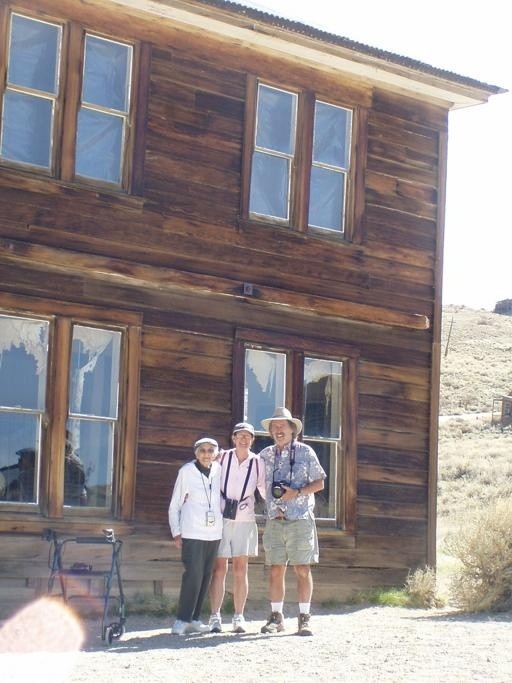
[35,522,132,649]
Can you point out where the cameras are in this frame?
[206,511,215,528]
[271,480,290,498]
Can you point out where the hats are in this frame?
[231,422,255,439]
[260,407,303,438]
[193,437,218,448]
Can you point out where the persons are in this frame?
[255,407,327,636]
[207,420,266,632]
[168,436,223,634]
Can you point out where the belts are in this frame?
[274,516,286,521]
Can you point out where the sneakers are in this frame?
[297,613,314,636]
[231,614,247,632]
[208,613,222,631]
[171,619,186,634]
[261,611,286,633]
[186,620,210,633]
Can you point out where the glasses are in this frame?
[199,448,215,454]
